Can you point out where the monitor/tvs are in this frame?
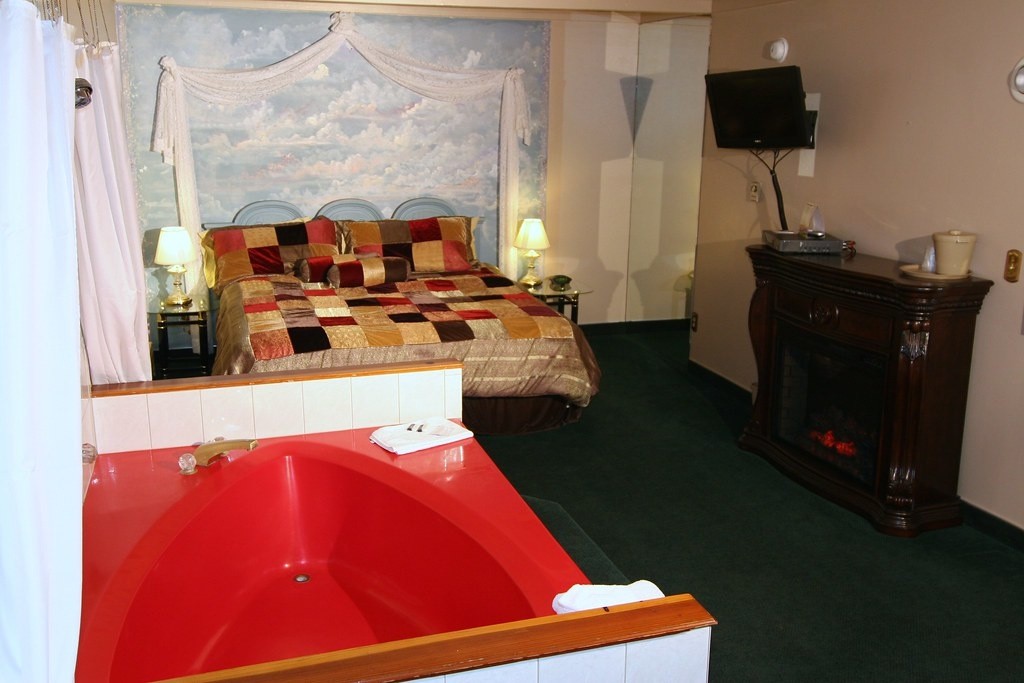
[704,65,811,150]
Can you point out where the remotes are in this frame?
[807,231,824,237]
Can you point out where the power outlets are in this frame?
[691,313,698,332]
[748,180,760,202]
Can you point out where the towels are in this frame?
[551,579,665,616]
[368,416,474,456]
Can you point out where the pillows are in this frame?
[352,239,471,277]
[295,253,379,283]
[335,215,481,273]
[216,242,340,287]
[327,257,411,287]
[204,216,334,284]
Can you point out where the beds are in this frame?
[204,198,602,439]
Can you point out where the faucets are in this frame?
[188,439,259,467]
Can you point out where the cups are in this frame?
[922,244,937,272]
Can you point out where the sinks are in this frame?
[76,442,566,683]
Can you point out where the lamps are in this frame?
[153,226,196,306]
[514,217,551,286]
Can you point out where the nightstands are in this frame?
[145,295,219,379]
[526,283,593,323]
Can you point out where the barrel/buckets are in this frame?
[930,229,978,275]
